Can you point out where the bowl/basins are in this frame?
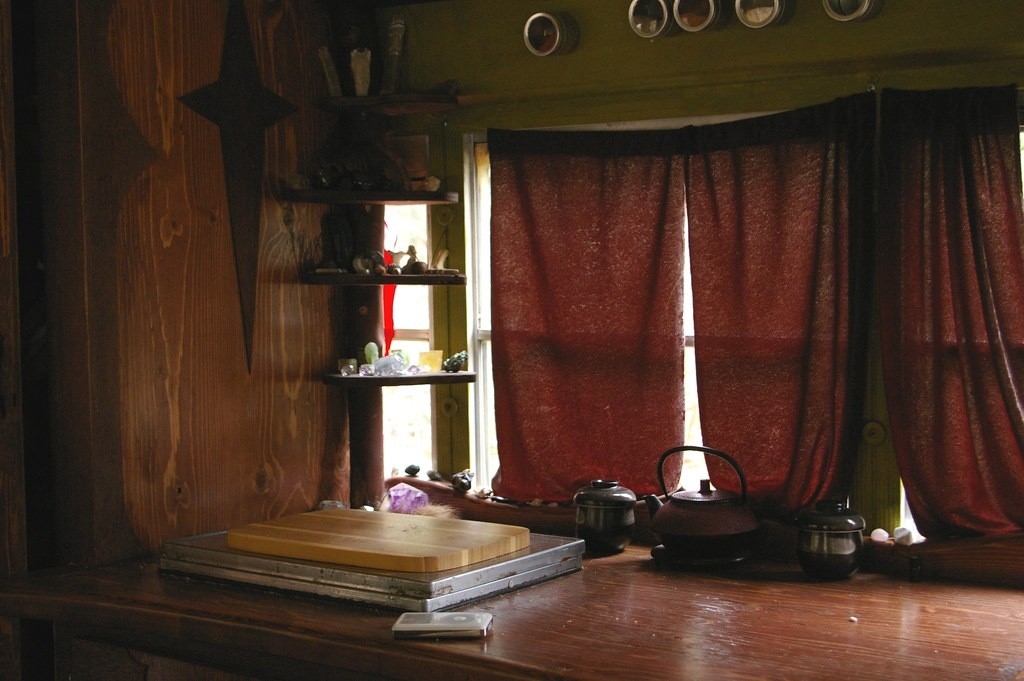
[573,479,637,555]
[796,497,867,580]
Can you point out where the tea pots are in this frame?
[642,445,766,564]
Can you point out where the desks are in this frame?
[0,554,1024,681]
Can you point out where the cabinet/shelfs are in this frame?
[273,89,476,390]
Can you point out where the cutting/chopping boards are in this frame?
[225,507,529,571]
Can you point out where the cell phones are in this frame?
[391,612,493,641]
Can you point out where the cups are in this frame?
[418,349,443,374]
[338,350,410,376]
